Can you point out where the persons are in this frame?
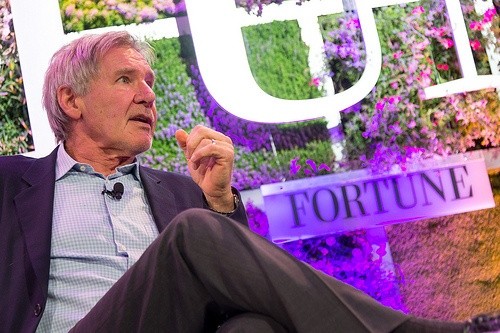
[0,30,500,332]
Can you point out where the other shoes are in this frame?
[462,314,500,333]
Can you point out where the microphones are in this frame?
[113,182,123,199]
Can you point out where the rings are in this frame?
[211,138,215,144]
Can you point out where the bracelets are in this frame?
[209,193,241,214]
